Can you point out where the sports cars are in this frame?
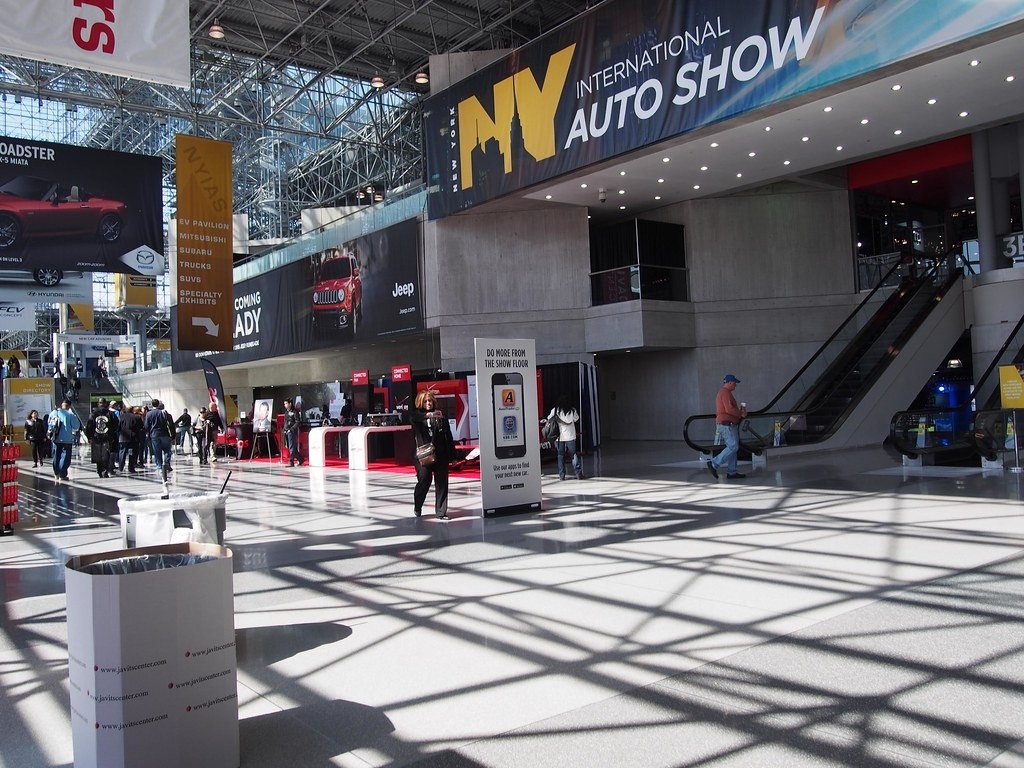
[0,176,127,249]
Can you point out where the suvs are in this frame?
[311,251,363,337]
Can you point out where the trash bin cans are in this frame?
[63,541,239,768]
[118,492,230,550]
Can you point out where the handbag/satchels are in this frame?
[417,442,438,465]
[191,418,197,436]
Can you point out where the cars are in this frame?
[216,422,253,454]
[0,269,84,287]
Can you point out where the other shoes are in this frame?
[164,465,173,471]
[203,459,208,465]
[59,476,68,480]
[436,515,450,519]
[578,475,585,480]
[55,474,60,480]
[414,505,421,517]
[286,463,294,468]
[96,462,145,477]
[32,462,36,469]
[40,459,43,467]
[296,459,305,466]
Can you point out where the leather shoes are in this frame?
[727,472,745,479]
[707,461,719,478]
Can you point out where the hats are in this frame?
[723,375,741,384]
[110,401,118,406]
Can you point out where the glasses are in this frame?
[199,411,205,414]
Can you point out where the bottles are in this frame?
[740,402,747,417]
[1,438,20,524]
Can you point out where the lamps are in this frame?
[159,115,166,126]
[2,93,6,101]
[234,98,240,107]
[388,59,397,73]
[271,67,278,78]
[66,99,72,111]
[416,67,428,84]
[114,107,121,118]
[371,71,384,88]
[252,84,256,94]
[209,17,225,38]
[15,91,21,103]
[274,115,284,123]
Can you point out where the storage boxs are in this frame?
[65,541,240,767]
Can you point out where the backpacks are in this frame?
[46,410,61,440]
[541,406,558,443]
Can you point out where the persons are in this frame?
[174,408,193,447]
[248,403,255,422]
[193,406,210,464]
[548,395,585,480]
[254,402,270,432]
[339,399,352,423]
[98,355,106,379]
[322,405,329,419]
[0,355,21,378]
[283,398,304,467]
[91,367,102,390]
[207,402,224,462]
[410,390,455,520]
[51,357,84,404]
[706,375,746,479]
[24,398,176,480]
